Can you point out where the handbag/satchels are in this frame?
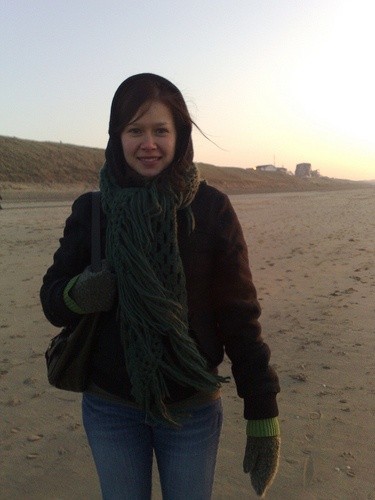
[44,312,106,393]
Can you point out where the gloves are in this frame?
[241,435,282,496]
[72,265,118,314]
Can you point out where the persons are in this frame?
[38,71,282,499]
